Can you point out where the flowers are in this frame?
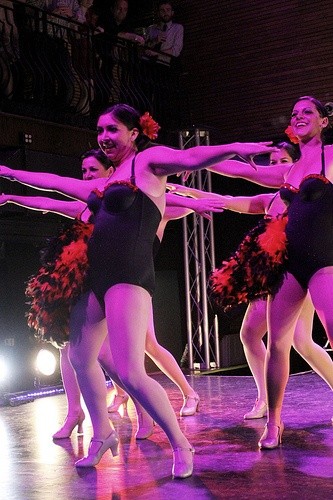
[284,125,300,143]
[139,112,160,140]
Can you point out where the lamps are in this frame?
[19,131,33,145]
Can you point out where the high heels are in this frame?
[135,411,157,439]
[244,398,267,419]
[179,393,201,416]
[257,421,284,448]
[172,446,195,478]
[108,393,129,413]
[75,430,119,468]
[52,411,85,439]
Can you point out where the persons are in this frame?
[0,149,226,439]
[206,96,333,449]
[108,195,234,416]
[0,103,280,478]
[165,140,333,418]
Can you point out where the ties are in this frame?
[150,25,166,61]
